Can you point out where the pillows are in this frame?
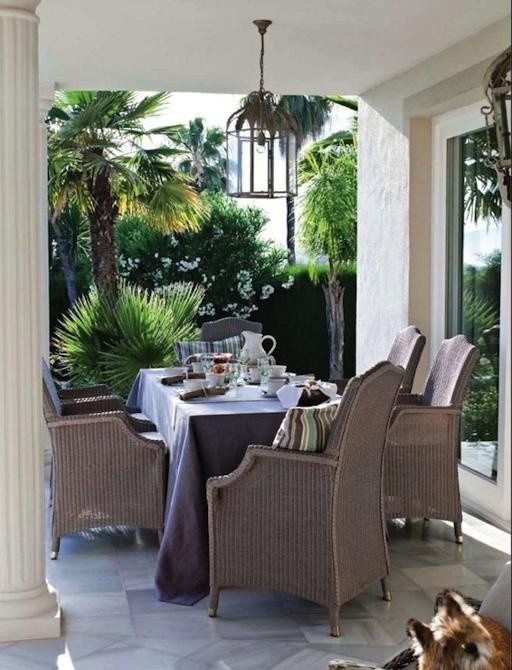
[179,337,243,367]
[271,401,343,456]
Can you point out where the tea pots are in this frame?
[239,331,277,365]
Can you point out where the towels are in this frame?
[179,386,225,401]
[184,352,224,364]
[162,370,205,385]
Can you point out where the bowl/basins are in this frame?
[183,379,211,393]
[284,381,330,407]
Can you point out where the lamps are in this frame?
[224,20,300,198]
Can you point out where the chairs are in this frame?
[43,379,170,561]
[386,332,478,545]
[203,317,263,339]
[43,360,152,420]
[388,326,424,393]
[206,358,401,637]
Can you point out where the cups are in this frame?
[192,361,247,387]
[249,357,287,397]
